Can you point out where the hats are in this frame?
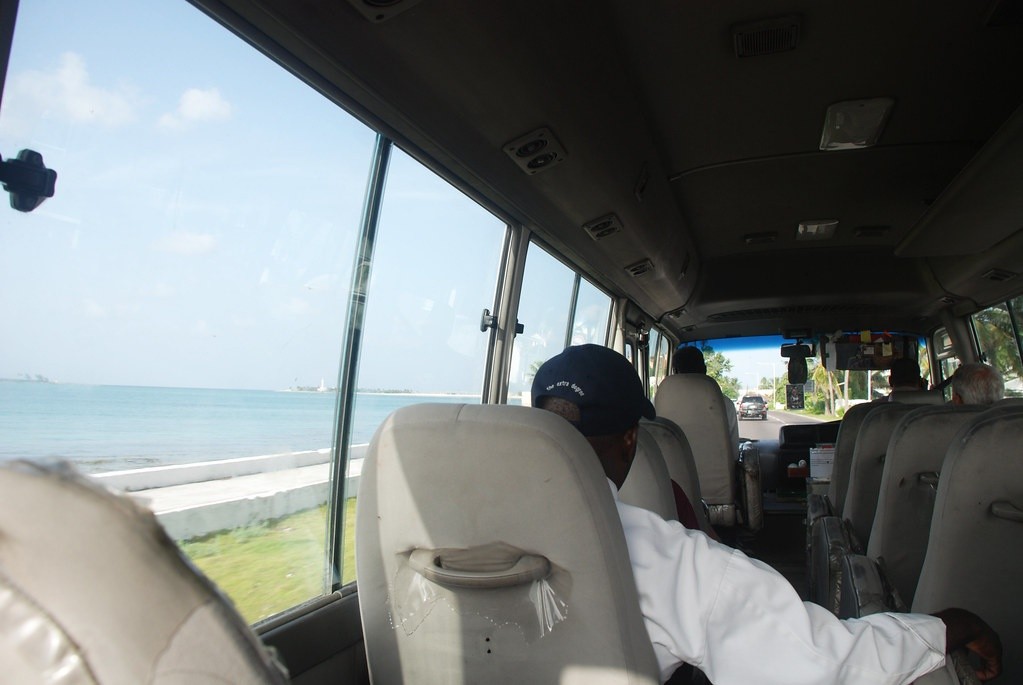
[531,344,656,436]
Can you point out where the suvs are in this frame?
[738,394,767,421]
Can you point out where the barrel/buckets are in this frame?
[810,448,835,477]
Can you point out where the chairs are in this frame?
[355,403,663,685]
[616,373,1023,685]
[1,457,294,685]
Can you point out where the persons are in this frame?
[650,346,741,463]
[530,343,1004,685]
[952,362,1003,407]
[871,356,922,402]
